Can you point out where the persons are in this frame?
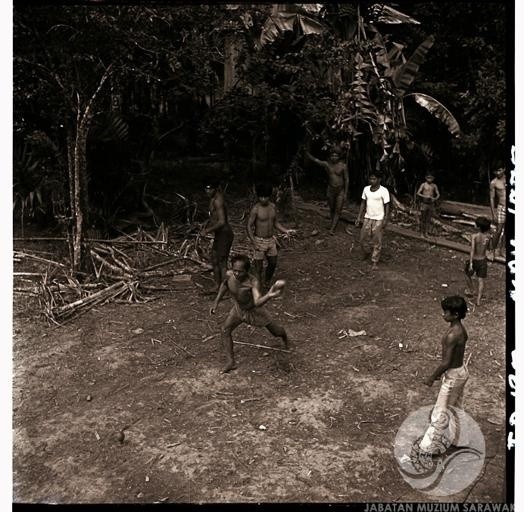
[486,164,507,263]
[303,145,351,238]
[244,183,296,296]
[415,171,441,239]
[207,254,296,375]
[463,216,491,307]
[353,168,391,272]
[413,294,469,457]
[195,177,235,301]
